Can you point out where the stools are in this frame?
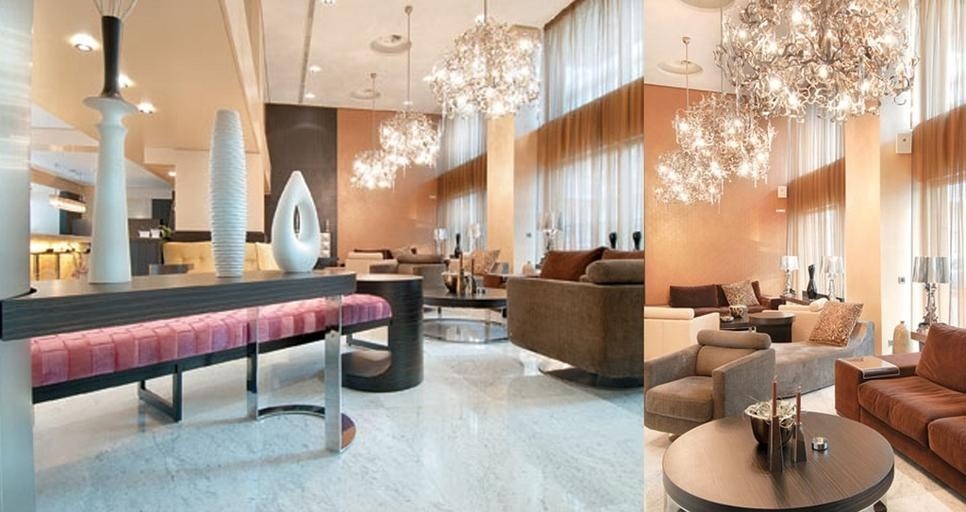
[368,260,446,291]
[772,321,875,399]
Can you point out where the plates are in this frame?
[721,317,734,321]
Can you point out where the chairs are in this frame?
[644,329,775,440]
[644,306,720,360]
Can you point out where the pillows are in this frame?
[539,246,603,281]
[670,279,759,307]
[810,301,865,347]
[601,249,644,259]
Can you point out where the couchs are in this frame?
[505,259,645,386]
[834,321,966,500]
[670,295,786,317]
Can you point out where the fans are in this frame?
[720,311,794,343]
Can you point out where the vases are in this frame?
[211,108,245,278]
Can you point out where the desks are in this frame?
[1,274,355,454]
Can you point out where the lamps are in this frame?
[469,222,482,253]
[821,255,844,302]
[432,227,448,255]
[69,32,155,114]
[351,0,544,190]
[914,256,950,329]
[779,255,800,293]
[49,188,87,213]
[651,0,921,210]
